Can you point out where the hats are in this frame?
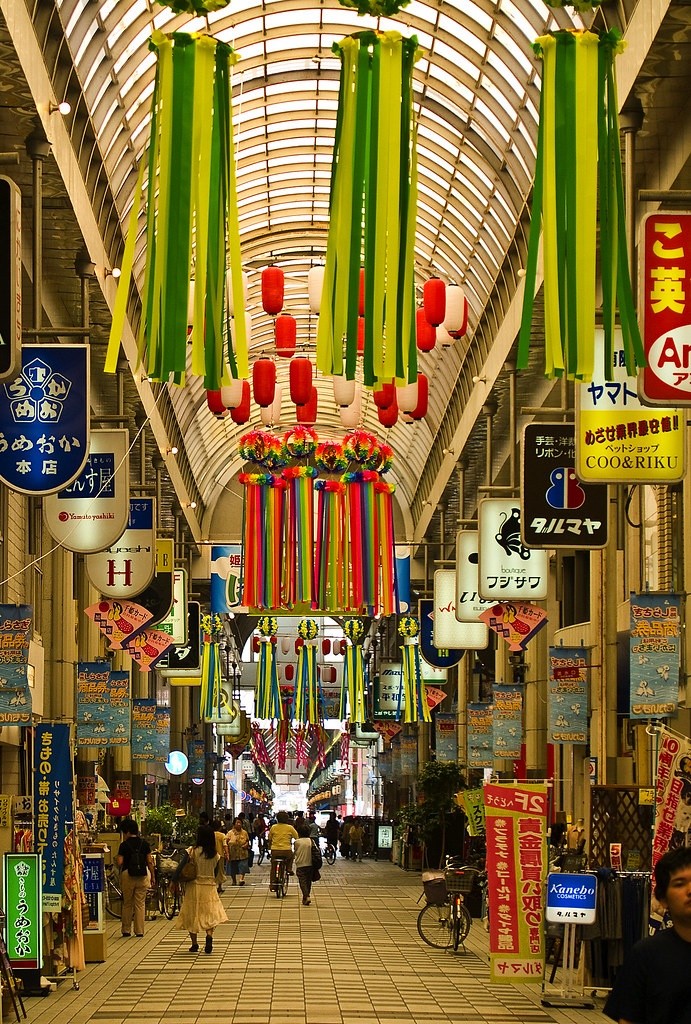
[297,825,310,836]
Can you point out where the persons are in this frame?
[602,846,691,1024]
[113,820,156,937]
[211,811,366,906]
[176,827,229,953]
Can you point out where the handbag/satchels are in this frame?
[248,850,254,867]
[178,847,196,881]
[310,839,322,869]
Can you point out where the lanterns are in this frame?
[204,267,468,428]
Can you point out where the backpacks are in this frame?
[126,839,146,876]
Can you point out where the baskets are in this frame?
[445,872,474,893]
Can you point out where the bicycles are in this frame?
[416,854,479,955]
[149,848,183,920]
[103,863,136,920]
[271,855,293,898]
[256,834,271,865]
[320,841,337,866]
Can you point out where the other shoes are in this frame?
[217,888,224,892]
[122,932,130,936]
[304,897,311,905]
[136,934,143,937]
[287,869,294,876]
[189,945,198,952]
[239,880,245,886]
[205,936,212,953]
